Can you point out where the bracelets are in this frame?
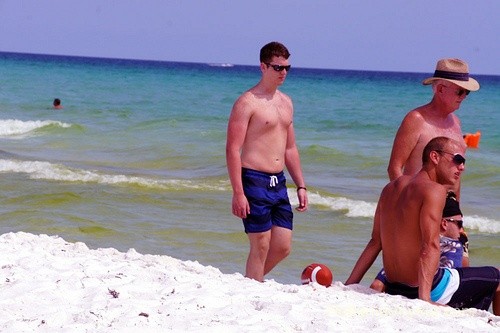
[297,187,306,192]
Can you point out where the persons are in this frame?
[370,58,500,320]
[226,42,308,281]
[54,99,63,108]
[344,137,500,312]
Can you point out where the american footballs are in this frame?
[300,264,332,288]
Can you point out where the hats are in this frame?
[442,198,463,217]
[423,58,480,92]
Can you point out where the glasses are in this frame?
[266,63,291,72]
[443,218,463,229]
[441,85,470,96]
[435,150,466,166]
